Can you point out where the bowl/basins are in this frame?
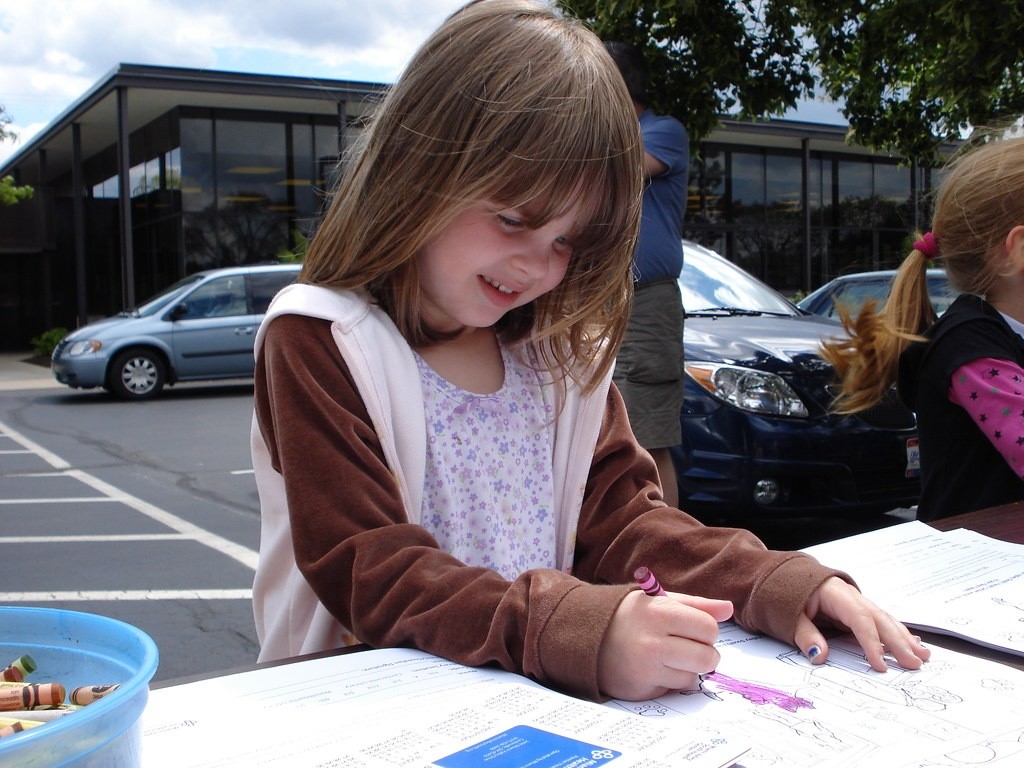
[0,606,160,768]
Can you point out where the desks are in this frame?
[148,502,1024,689]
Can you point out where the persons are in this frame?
[602,43,690,514]
[821,136,1024,522]
[248,0,931,704]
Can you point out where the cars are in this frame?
[50,261,307,402]
[676,240,928,547]
[792,264,985,325]
